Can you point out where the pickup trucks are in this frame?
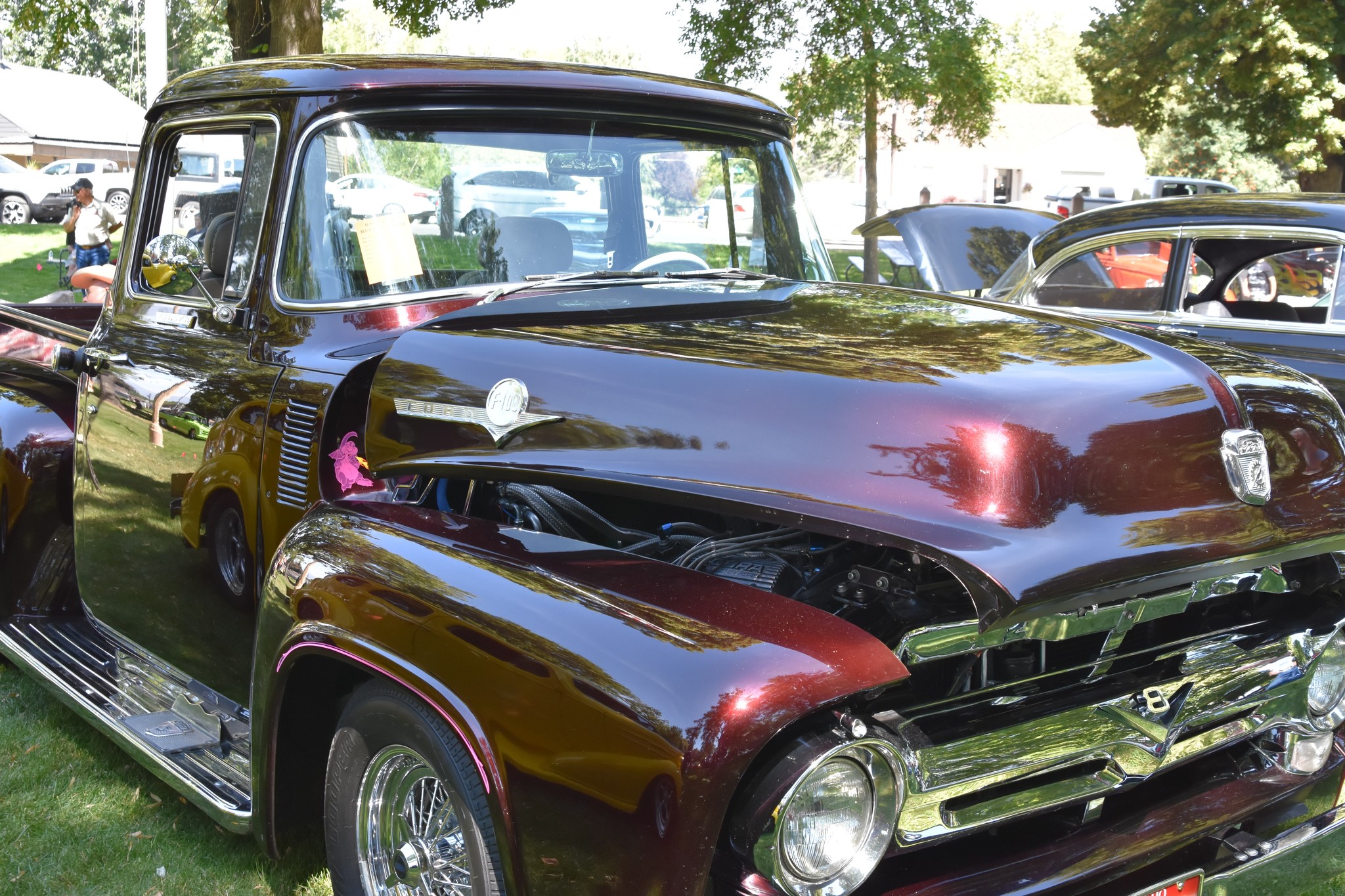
[1043,172,1239,224]
[0,49,1344,895]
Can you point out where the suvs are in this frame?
[710,180,758,240]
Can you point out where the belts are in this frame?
[78,241,106,250]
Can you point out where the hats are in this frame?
[70,178,93,190]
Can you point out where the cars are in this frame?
[848,191,1345,413]
[1089,239,1170,290]
[0,153,665,241]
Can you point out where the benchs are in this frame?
[848,255,889,285]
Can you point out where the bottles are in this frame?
[48,250,53,262]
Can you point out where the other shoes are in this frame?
[59,276,70,284]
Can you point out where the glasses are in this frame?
[75,190,81,193]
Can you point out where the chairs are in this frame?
[1187,299,1232,318]
[454,215,573,287]
[184,210,347,302]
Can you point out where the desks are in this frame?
[46,259,66,270]
[877,240,923,289]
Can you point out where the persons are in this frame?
[131,397,150,413]
[148,258,163,265]
[62,178,123,297]
[70,264,118,305]
[185,213,203,239]
[60,243,78,286]
